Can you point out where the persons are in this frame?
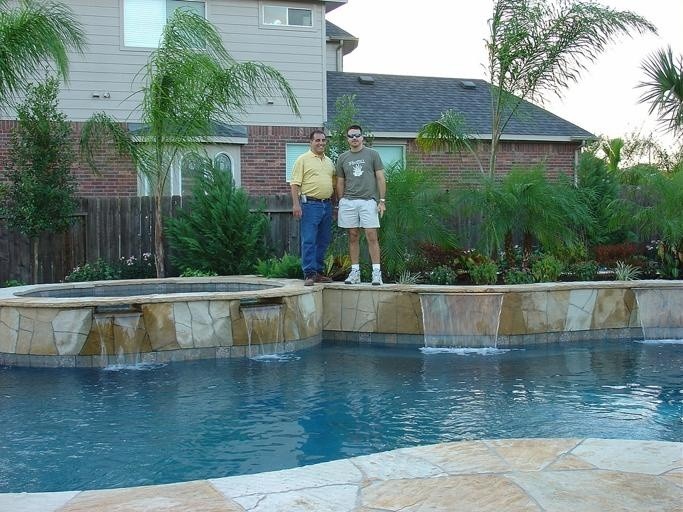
[290,131,336,286]
[336,125,386,284]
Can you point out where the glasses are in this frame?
[348,134,361,138]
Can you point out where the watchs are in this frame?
[379,199,386,202]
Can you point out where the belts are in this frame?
[307,198,332,202]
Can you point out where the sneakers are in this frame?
[344,270,360,284]
[372,271,383,285]
[304,273,332,286]
